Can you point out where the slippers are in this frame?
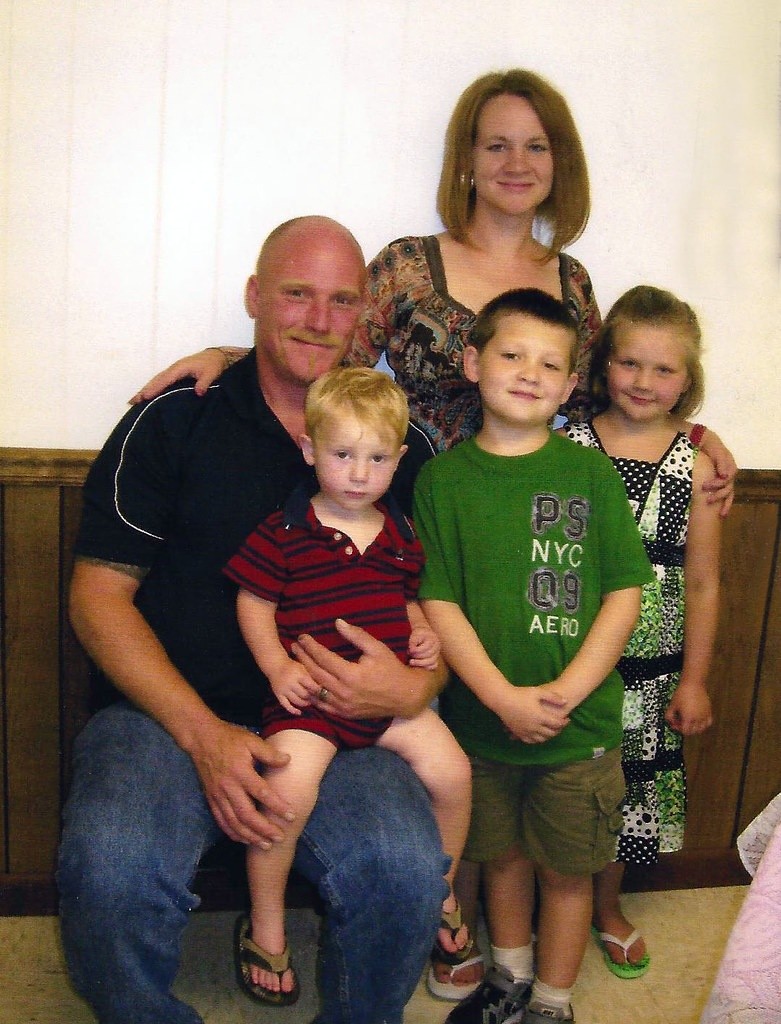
[427,953,483,1000]
[235,917,300,1006]
[592,923,650,979]
[431,897,474,963]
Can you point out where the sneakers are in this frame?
[524,1003,576,1024]
[445,963,534,1024]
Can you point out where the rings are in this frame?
[319,688,328,700]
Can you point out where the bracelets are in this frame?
[203,348,230,376]
[689,423,707,446]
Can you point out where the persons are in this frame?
[414,288,657,1024]
[55,216,452,1024]
[129,67,739,1000]
[551,285,722,977]
[222,366,472,1006]
